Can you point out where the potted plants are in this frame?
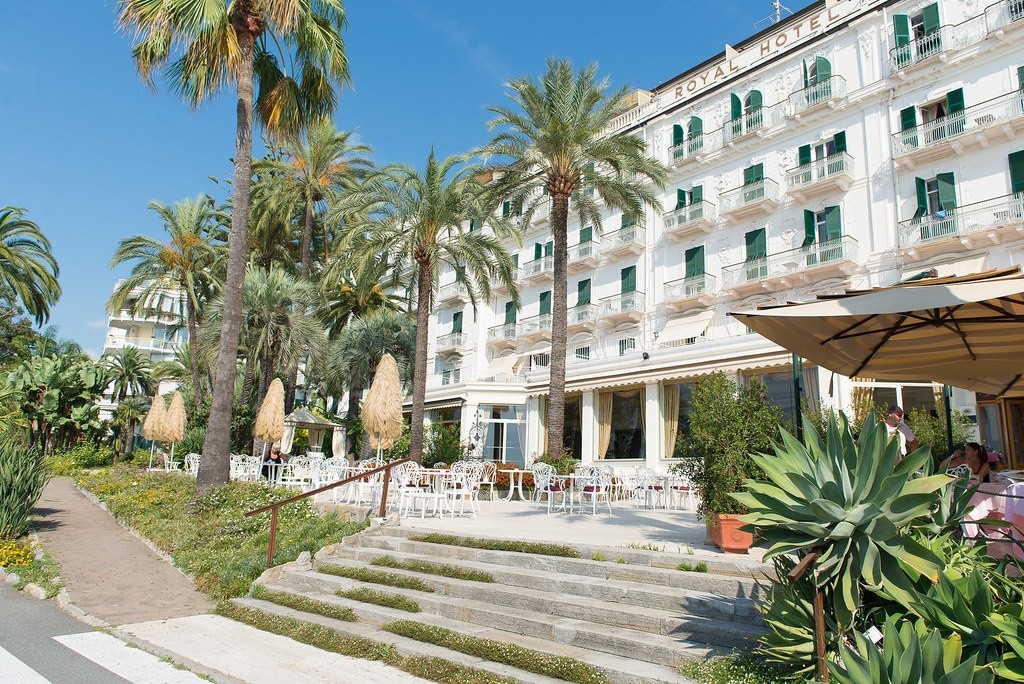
[667,373,760,555]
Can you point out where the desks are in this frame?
[955,478,1024,563]
[255,454,694,520]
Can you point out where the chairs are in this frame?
[182,450,699,523]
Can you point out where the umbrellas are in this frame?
[726,263,1024,398]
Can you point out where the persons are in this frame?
[262,441,290,481]
[347,441,360,461]
[939,442,990,484]
[876,405,919,459]
